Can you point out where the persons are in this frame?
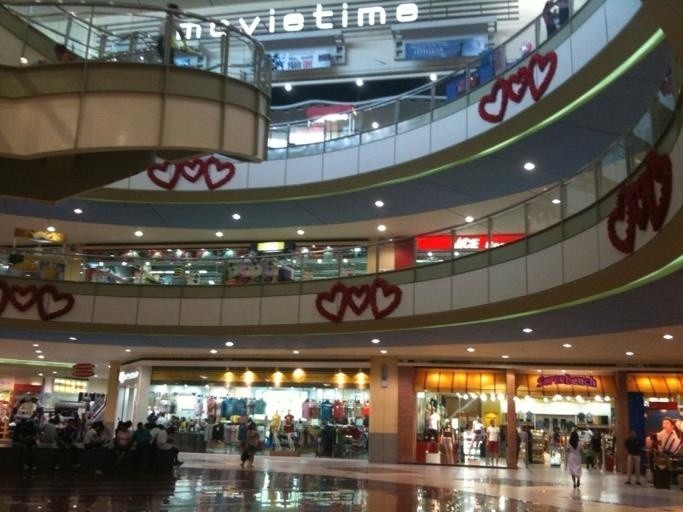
[649,434,659,472]
[423,407,442,454]
[0,410,203,480]
[467,415,486,461]
[440,423,456,464]
[554,0,569,26]
[486,419,500,467]
[645,416,683,457]
[280,410,296,452]
[458,427,467,464]
[515,426,615,489]
[236,416,261,470]
[37,42,73,62]
[624,430,643,485]
[267,410,283,451]
[154,4,188,65]
[543,0,558,39]
